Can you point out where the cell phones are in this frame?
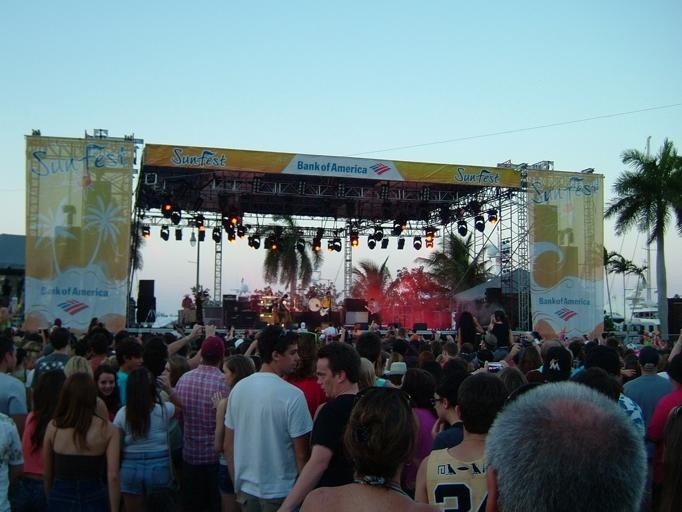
[154,377,163,388]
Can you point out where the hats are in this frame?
[201,335,225,359]
[639,346,659,368]
[542,347,572,382]
[385,362,407,375]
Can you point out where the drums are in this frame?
[302,298,321,314]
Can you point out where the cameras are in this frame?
[488,362,504,374]
[197,326,206,336]
[517,333,531,348]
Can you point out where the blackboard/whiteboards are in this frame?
[345,311,369,324]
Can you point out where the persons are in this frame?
[1,287,682,511]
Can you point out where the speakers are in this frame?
[667,297,682,337]
[222,294,236,328]
[328,312,340,321]
[413,323,427,333]
[486,288,502,307]
[344,298,368,311]
[137,279,156,323]
[290,311,321,328]
[240,310,256,324]
